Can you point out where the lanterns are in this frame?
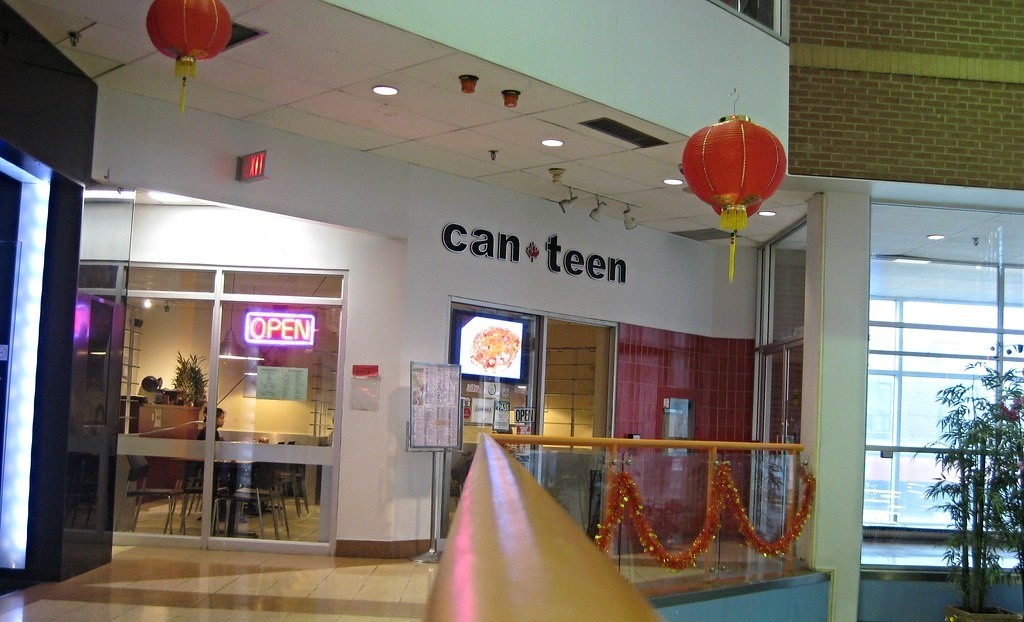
[145,0,232,115]
[682,114,787,279]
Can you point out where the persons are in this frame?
[179,407,247,522]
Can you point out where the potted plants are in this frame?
[924,353,1024,622]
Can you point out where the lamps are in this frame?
[622,204,638,231]
[560,187,579,213]
[589,195,607,222]
[220,275,265,362]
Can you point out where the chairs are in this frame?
[121,440,309,543]
